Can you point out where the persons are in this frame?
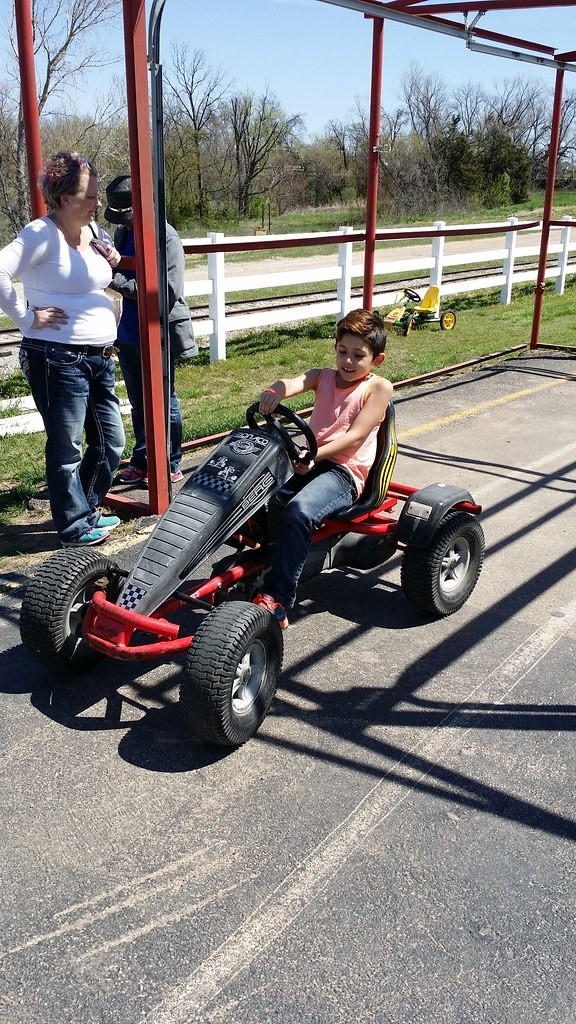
[237,307,395,629]
[103,174,203,485]
[0,153,125,546]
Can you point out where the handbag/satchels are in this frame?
[104,286,123,328]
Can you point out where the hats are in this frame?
[105,175,134,225]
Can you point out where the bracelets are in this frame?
[265,388,279,395]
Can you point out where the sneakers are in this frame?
[251,594,289,629]
[61,528,109,546]
[96,515,121,530]
[142,470,184,485]
[117,466,146,482]
